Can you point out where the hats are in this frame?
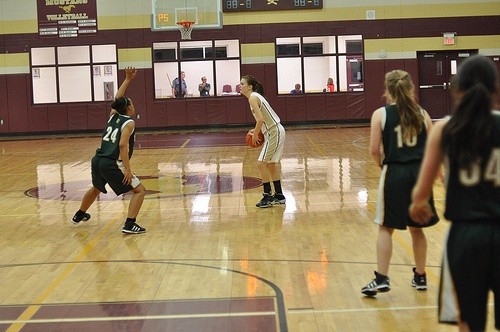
[202,77,207,80]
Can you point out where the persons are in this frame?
[327,78,334,92]
[239,75,286,208]
[361,70,442,297]
[409,55,500,332]
[71,65,146,234]
[171,71,187,98]
[198,77,211,97]
[290,84,302,94]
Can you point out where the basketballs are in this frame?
[246,130,264,148]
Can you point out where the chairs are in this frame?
[223,85,231,92]
[236,85,240,93]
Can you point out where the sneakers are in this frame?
[273,193,285,203]
[362,271,390,295]
[122,220,146,233]
[411,268,427,290]
[71,213,90,225]
[256,193,275,207]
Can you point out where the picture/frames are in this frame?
[33,69,40,77]
[93,67,100,75]
[104,66,112,74]
